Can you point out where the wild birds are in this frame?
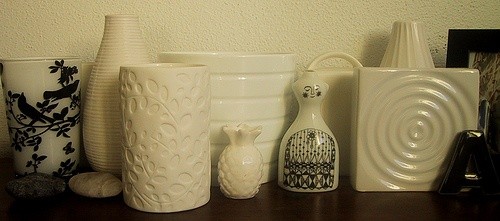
[43,80,79,103]
[18,92,56,126]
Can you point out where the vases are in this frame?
[159,52,294,185]
[119,65,212,212]
[378,20,435,69]
[82,14,151,175]
[217,122,263,199]
[0,56,80,179]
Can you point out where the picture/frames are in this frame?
[445,29,500,111]
